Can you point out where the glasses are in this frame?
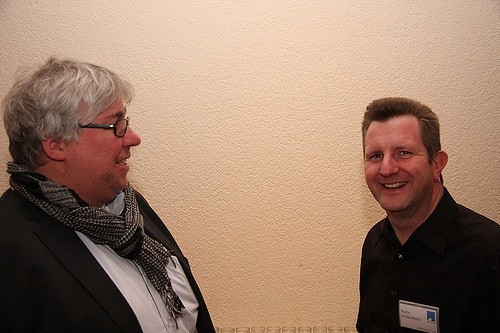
[79,116,130,137]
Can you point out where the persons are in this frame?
[0,59,215,333]
[355,96,499,333]
